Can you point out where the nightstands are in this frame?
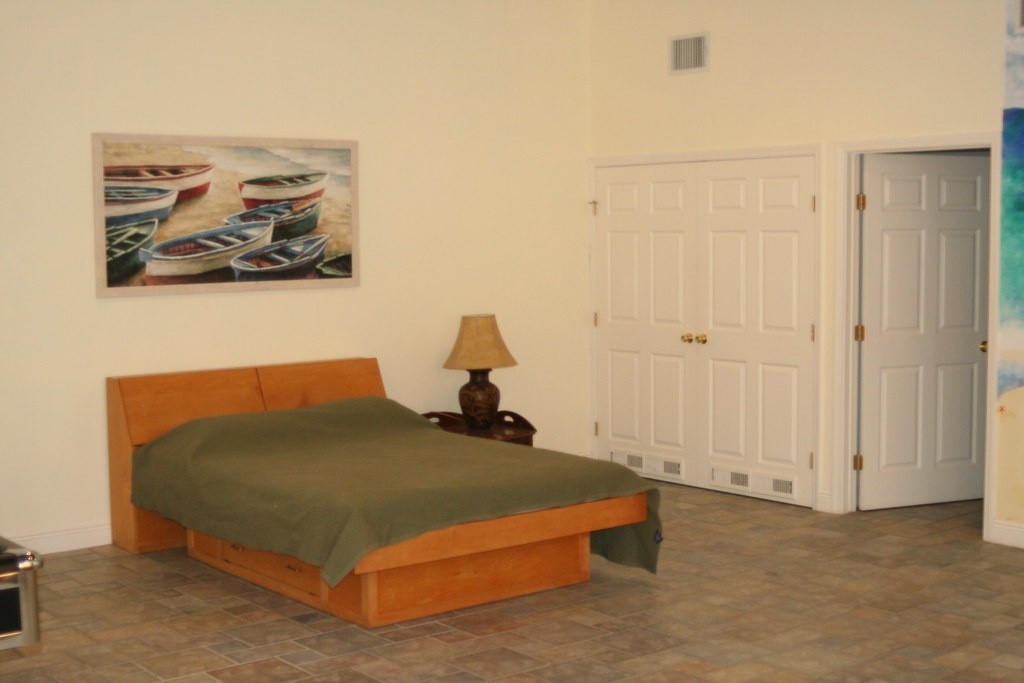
[437,411,536,449]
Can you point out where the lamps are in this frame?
[441,313,518,431]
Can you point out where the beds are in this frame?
[106,357,663,629]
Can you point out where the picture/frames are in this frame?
[92,132,360,297]
